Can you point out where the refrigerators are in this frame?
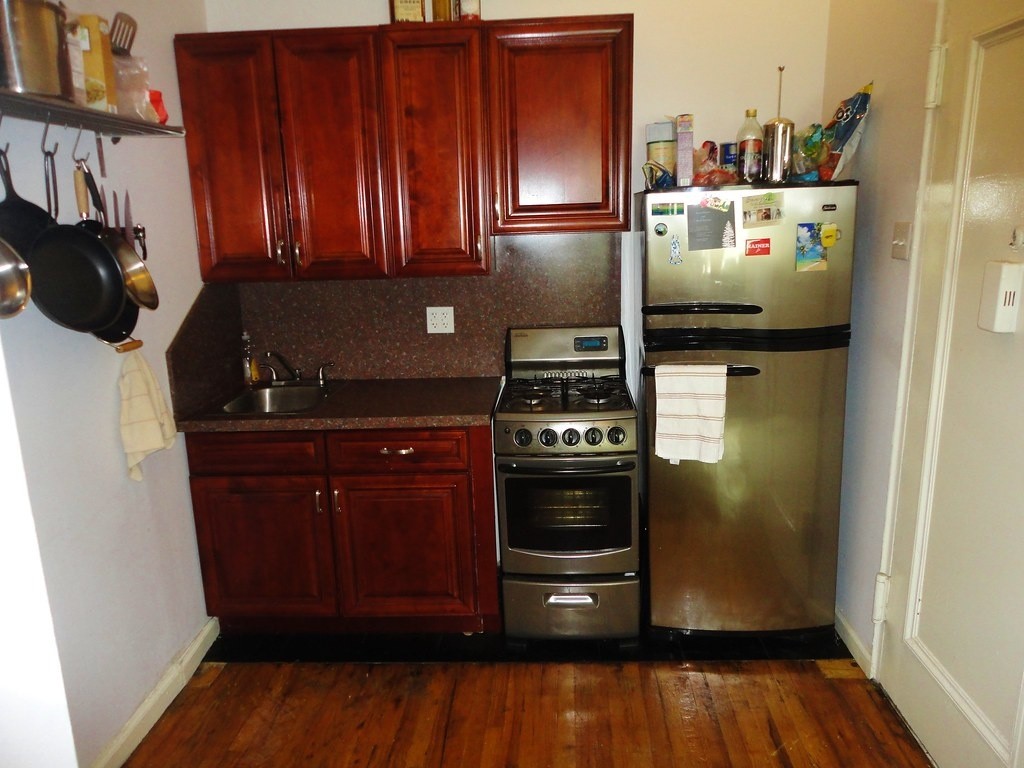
[636,182,857,633]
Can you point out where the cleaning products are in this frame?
[240,331,261,386]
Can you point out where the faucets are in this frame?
[261,350,301,380]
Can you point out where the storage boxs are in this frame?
[675,113,693,187]
[81,13,120,112]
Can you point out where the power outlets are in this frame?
[426,307,455,334]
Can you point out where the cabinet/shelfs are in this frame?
[173,25,387,285]
[376,21,496,281]
[485,14,635,235]
[184,424,502,637]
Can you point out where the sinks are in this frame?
[215,387,328,416]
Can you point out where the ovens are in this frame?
[494,456,642,639]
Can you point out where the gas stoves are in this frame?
[491,325,639,454]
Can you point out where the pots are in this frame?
[0,145,159,353]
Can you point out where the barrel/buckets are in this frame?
[1,0,67,97]
[111,47,159,124]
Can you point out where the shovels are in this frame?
[110,11,138,57]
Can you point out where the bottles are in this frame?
[431,0,480,21]
[737,109,764,185]
[389,1,425,22]
